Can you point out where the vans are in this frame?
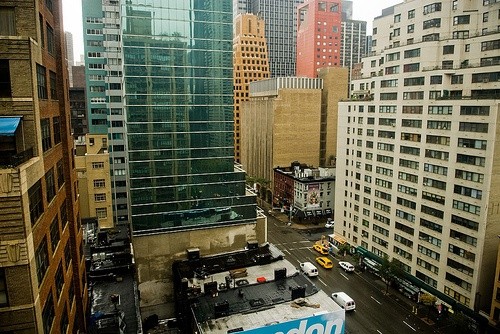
[331,291,355,312]
[300,262,319,278]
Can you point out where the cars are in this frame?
[325,222,335,229]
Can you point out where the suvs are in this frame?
[313,244,329,255]
[316,256,333,268]
[339,261,354,272]
